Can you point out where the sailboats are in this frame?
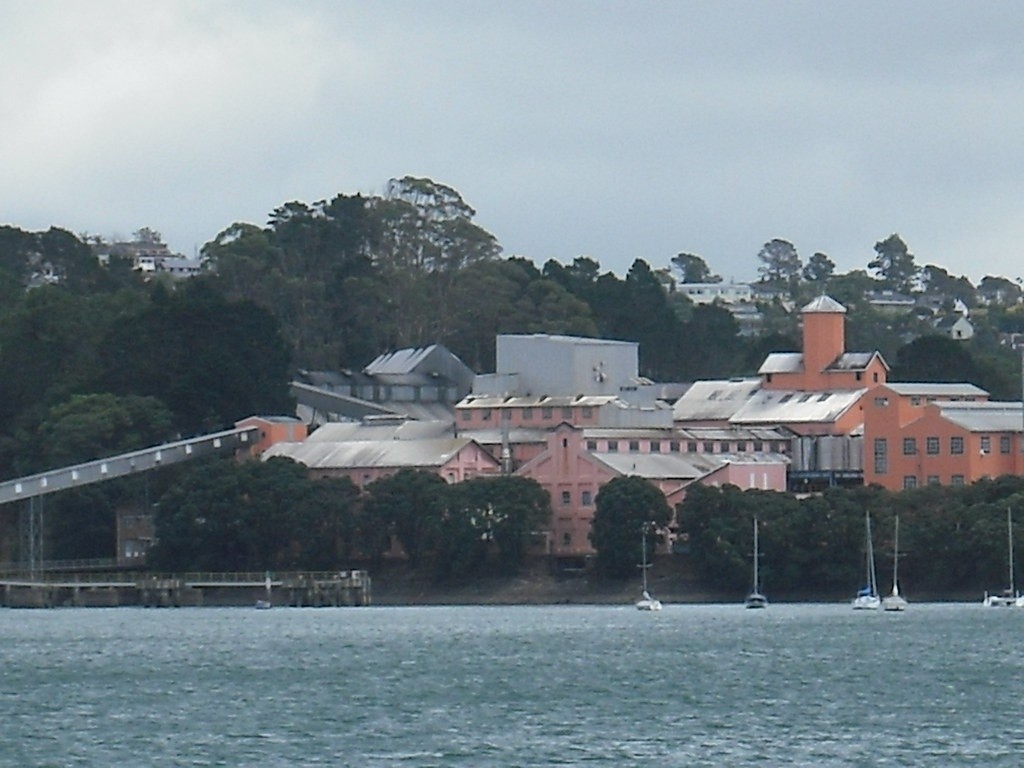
[636,533,662,611]
[745,518,769,609]
[882,515,910,611]
[852,509,881,611]
[983,506,1024,609]
